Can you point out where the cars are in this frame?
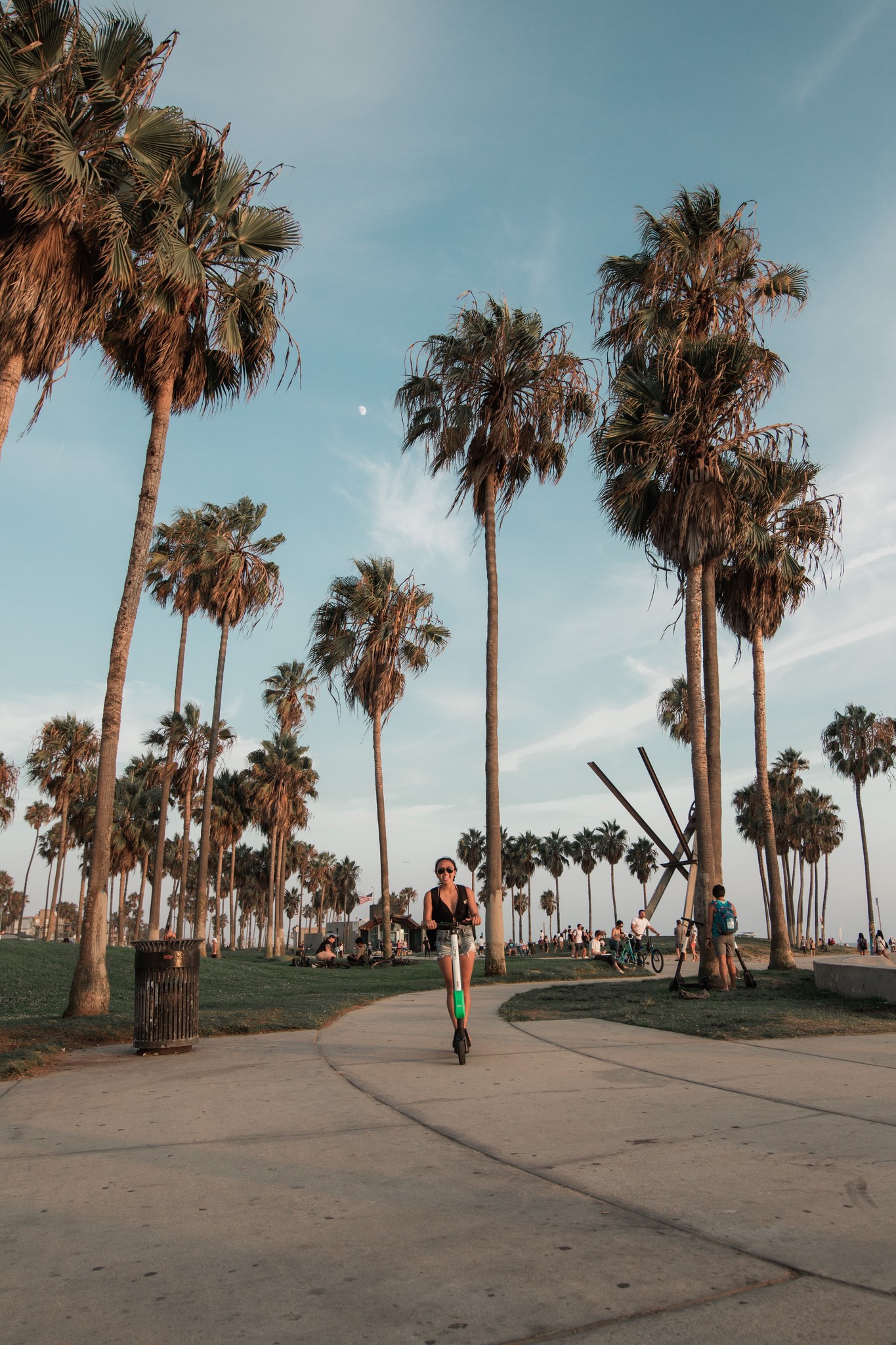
[0,932,34,942]
[374,939,397,951]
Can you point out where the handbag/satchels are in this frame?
[884,939,889,949]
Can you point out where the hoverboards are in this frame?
[669,917,713,992]
[734,943,758,987]
[437,921,470,1066]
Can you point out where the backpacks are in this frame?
[167,932,175,940]
[712,901,738,935]
[889,939,893,945]
[859,938,867,950]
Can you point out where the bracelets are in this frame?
[706,937,710,940]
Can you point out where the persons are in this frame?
[673,920,700,962]
[705,884,737,992]
[300,920,636,974]
[227,944,231,947]
[210,938,218,958]
[624,910,660,967]
[164,925,176,943]
[800,930,896,962]
[422,858,481,1032]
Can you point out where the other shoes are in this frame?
[616,955,620,957]
[674,957,680,961]
[629,967,636,972]
[616,969,625,974]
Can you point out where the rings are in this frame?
[705,945,706,946]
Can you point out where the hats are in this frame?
[213,938,217,943]
[326,935,336,939]
[368,942,371,944]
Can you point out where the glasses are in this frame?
[618,925,623,927]
[437,868,455,875]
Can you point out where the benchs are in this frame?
[504,945,531,956]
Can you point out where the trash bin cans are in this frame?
[129,938,204,1054]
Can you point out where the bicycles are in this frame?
[287,943,350,969]
[819,943,831,955]
[289,947,295,955]
[891,943,895,953]
[614,931,645,967]
[336,948,340,958]
[627,934,664,973]
[370,957,421,970]
